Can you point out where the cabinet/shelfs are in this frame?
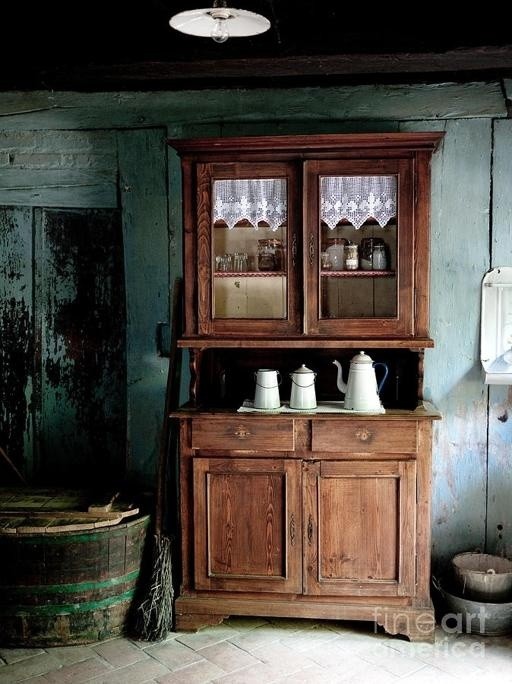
[162,130,450,643]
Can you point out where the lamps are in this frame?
[169,2,273,44]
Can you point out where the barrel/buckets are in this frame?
[0,490,155,648]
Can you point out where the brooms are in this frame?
[132,278,183,642]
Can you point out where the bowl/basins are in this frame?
[432,547,512,638]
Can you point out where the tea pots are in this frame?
[333,351,388,412]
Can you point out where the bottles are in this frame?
[258,237,283,270]
[231,253,248,271]
[321,237,387,271]
[255,369,281,410]
[288,364,319,410]
